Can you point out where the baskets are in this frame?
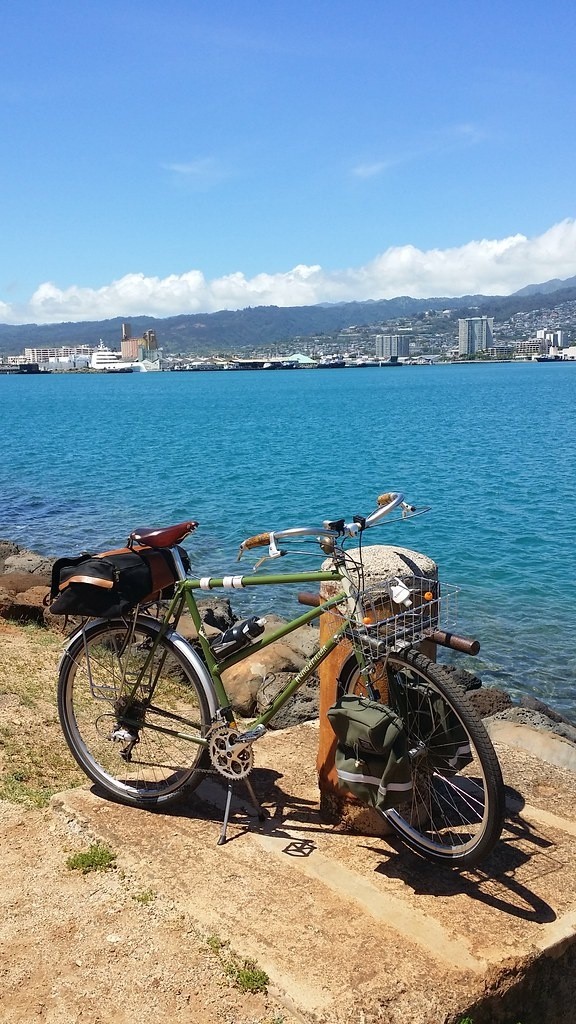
[320,574,461,659]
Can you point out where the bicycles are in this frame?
[56,490,508,871]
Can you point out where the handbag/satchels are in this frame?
[42,542,190,619]
[389,667,473,776]
[325,694,413,810]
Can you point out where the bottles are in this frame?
[211,616,268,658]
